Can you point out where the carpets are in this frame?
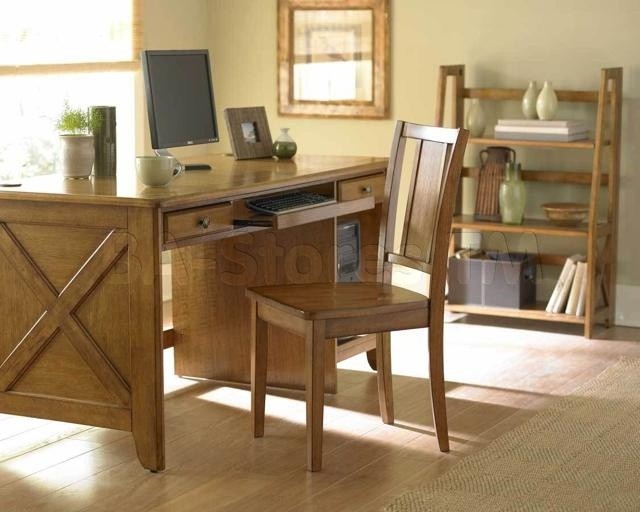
[380,354,640,512]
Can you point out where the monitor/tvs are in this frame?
[141,47,220,175]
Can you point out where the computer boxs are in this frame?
[336,218,361,343]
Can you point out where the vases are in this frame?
[522,79,558,120]
[499,162,525,223]
[467,98,486,137]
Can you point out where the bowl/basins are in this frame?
[542,203,593,230]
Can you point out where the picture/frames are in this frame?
[227,108,274,160]
[275,0,393,121]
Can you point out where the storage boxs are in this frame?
[449,248,536,309]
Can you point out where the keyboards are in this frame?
[244,189,338,215]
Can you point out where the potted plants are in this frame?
[53,99,105,180]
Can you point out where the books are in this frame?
[493,116,592,143]
[545,251,586,316]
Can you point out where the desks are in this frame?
[0,157,389,471]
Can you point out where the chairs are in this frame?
[247,120,470,473]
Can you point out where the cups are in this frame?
[92,107,115,177]
[136,157,182,187]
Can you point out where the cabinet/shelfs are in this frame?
[437,65,624,339]
